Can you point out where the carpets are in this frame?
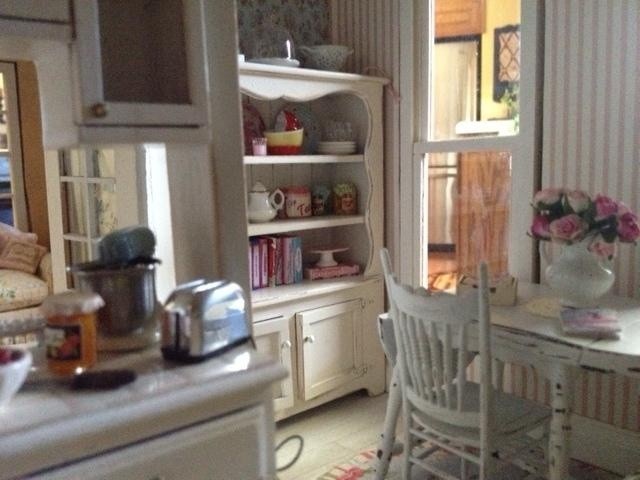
[316,430,626,478]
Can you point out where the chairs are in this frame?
[0,221,53,346]
[371,243,554,479]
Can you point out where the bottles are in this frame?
[41,292,103,379]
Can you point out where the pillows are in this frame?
[1,236,50,275]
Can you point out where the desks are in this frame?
[438,274,640,480]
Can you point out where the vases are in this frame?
[543,243,617,306]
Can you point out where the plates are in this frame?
[248,56,300,65]
[241,103,267,155]
[316,140,359,155]
[275,104,321,153]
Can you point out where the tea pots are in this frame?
[247,180,284,224]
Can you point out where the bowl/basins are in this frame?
[0,344,31,412]
[266,125,306,154]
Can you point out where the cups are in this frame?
[281,185,313,217]
[253,137,268,156]
[64,256,160,333]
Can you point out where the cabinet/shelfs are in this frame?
[1,341,291,479]
[236,61,395,424]
[39,0,215,150]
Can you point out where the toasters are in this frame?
[157,277,251,364]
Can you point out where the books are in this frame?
[248,235,304,291]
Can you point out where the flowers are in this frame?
[524,189,640,266]
